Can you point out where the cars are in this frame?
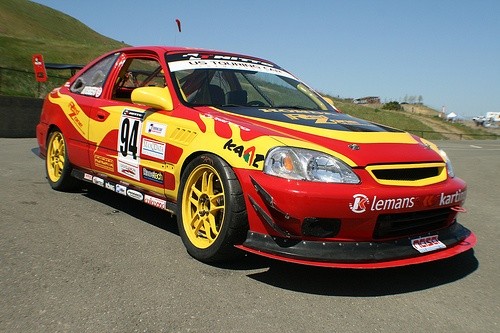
[29,43,479,273]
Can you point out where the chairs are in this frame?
[198,84,226,107]
